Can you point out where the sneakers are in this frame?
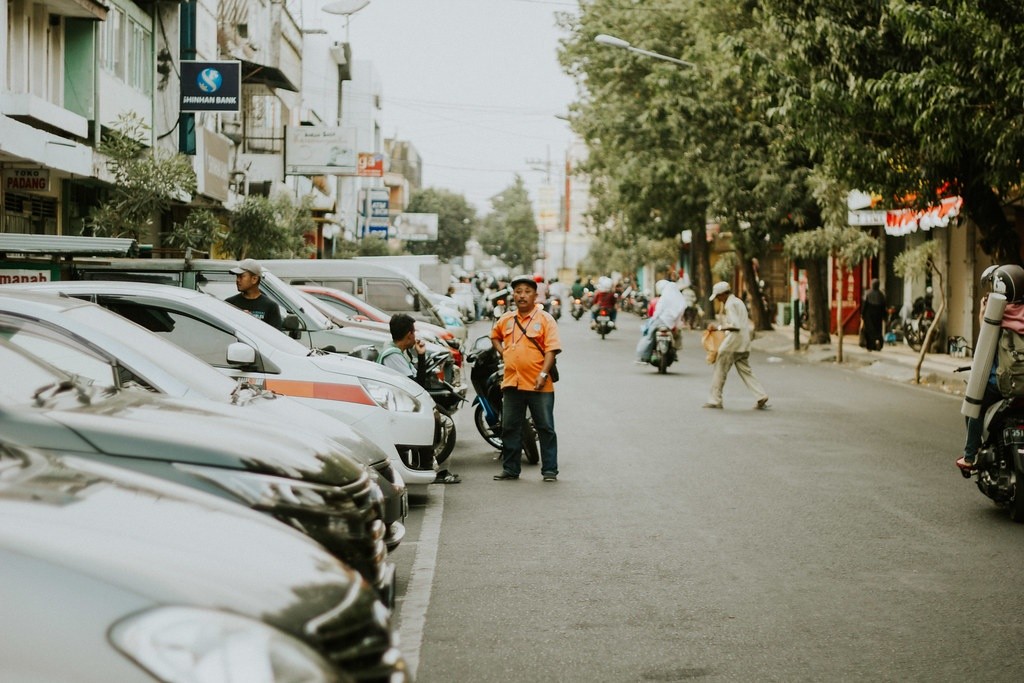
[432,469,461,483]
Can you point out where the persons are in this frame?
[572,275,618,330]
[621,278,686,363]
[860,279,890,351]
[488,277,562,482]
[377,313,462,484]
[682,287,698,330]
[223,259,282,333]
[447,271,561,322]
[956,264,1024,470]
[700,281,769,409]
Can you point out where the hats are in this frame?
[510,277,537,291]
[709,282,730,302]
[228,258,262,277]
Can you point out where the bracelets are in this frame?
[539,371,548,380]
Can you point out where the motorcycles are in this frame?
[948,365,1024,518]
[547,300,563,324]
[446,304,542,465]
[571,298,585,319]
[648,324,680,374]
[594,310,616,339]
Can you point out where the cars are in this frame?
[0,313,404,610]
[1,438,416,683]
[1,281,461,485]
[0,288,409,550]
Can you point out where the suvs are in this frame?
[0,232,482,412]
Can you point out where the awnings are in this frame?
[0,113,193,204]
[236,58,298,95]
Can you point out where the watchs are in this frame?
[718,325,722,331]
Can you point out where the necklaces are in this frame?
[507,311,538,352]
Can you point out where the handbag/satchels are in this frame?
[548,359,560,382]
[700,323,724,363]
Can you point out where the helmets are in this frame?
[989,265,1024,304]
[535,276,543,282]
[655,280,669,296]
[575,277,582,283]
[598,276,613,289]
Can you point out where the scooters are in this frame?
[898,287,941,353]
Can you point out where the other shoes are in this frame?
[493,471,519,480]
[543,474,556,481]
[704,401,723,407]
[956,456,973,471]
[754,396,769,408]
